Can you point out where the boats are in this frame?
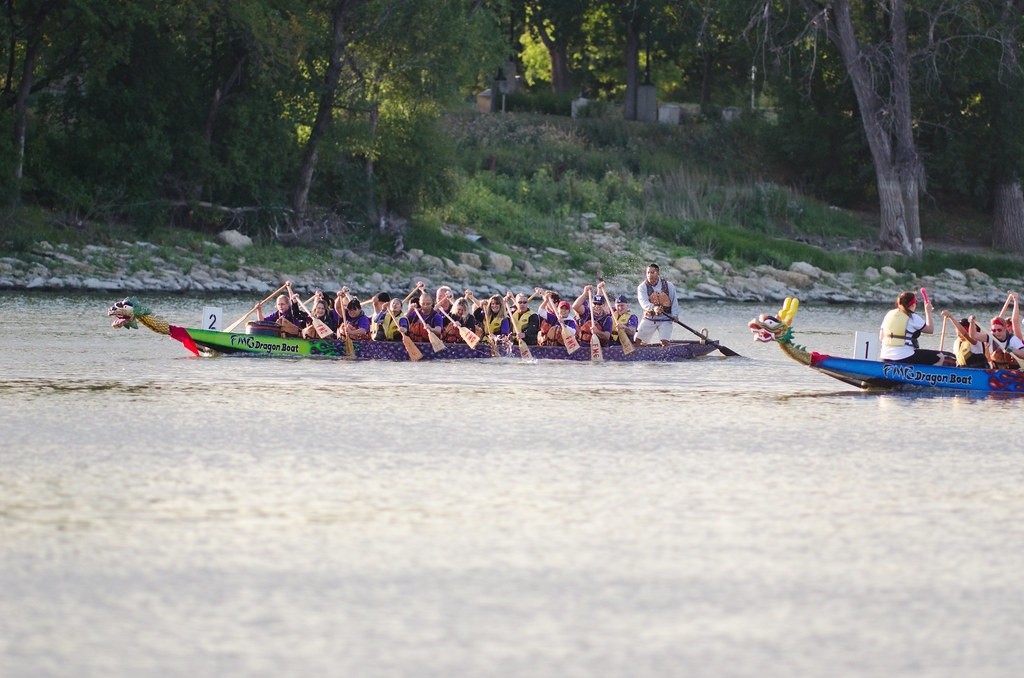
[107,295,719,360]
[748,297,1024,392]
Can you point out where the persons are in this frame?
[292,286,371,340]
[968,290,1024,371]
[878,291,934,364]
[507,287,579,346]
[572,282,638,344]
[941,310,987,368]
[255,280,293,322]
[632,263,679,346]
[373,281,510,344]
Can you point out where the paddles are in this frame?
[299,291,320,308]
[223,283,290,333]
[402,284,423,304]
[503,292,511,300]
[340,295,354,355]
[972,315,1024,369]
[939,313,949,352]
[527,291,539,302]
[385,305,424,361]
[295,297,333,339]
[465,292,473,298]
[435,291,452,307]
[547,295,581,356]
[598,281,636,355]
[658,305,741,357]
[412,306,446,353]
[586,285,604,361]
[482,304,499,356]
[505,300,533,360]
[360,300,373,306]
[438,307,481,349]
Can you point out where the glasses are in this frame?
[420,301,433,308]
[516,301,528,305]
[593,302,603,305]
[992,327,1003,333]
[490,302,500,305]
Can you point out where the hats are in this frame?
[559,301,570,310]
[615,295,628,304]
[593,295,606,304]
